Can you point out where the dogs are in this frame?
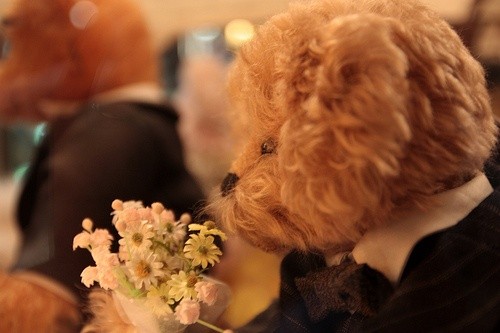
[199,0,499,329]
[1,1,222,332]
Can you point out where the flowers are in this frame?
[72,199,234,333]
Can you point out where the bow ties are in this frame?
[294,253,371,317]
